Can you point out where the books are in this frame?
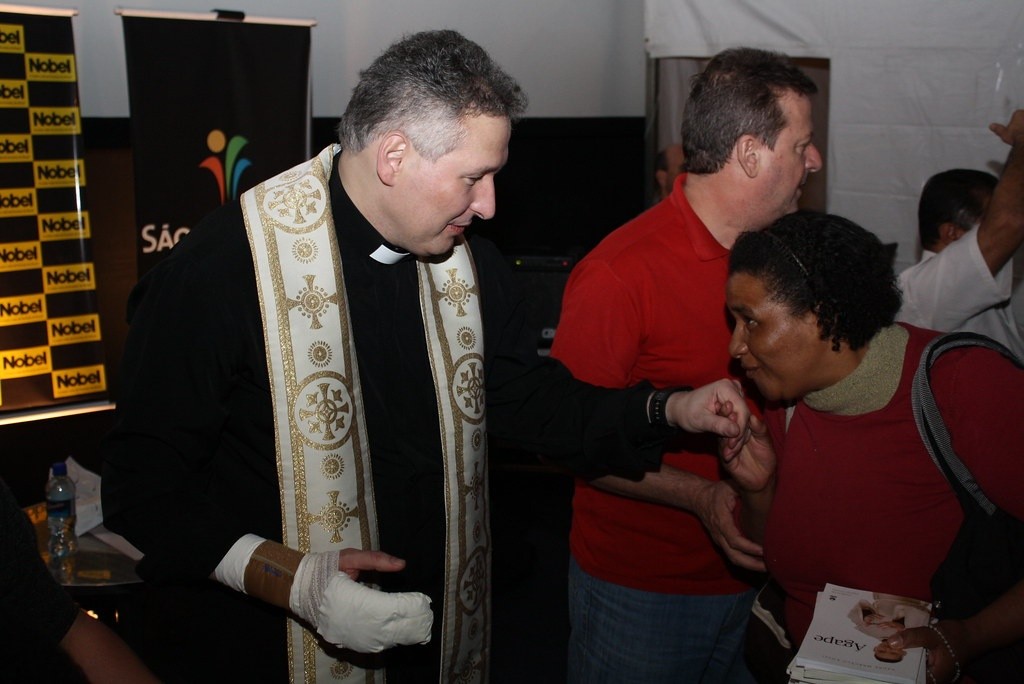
[785,583,939,684]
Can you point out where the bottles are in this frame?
[46,462,79,559]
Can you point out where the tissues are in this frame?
[49,457,103,537]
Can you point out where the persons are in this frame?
[550,44,822,684]
[893,109,1024,360]
[716,207,1024,684]
[848,592,930,663]
[0,477,161,684]
[100,30,750,684]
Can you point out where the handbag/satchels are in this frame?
[911,332,1024,684]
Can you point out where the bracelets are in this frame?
[648,385,694,426]
[925,624,960,684]
